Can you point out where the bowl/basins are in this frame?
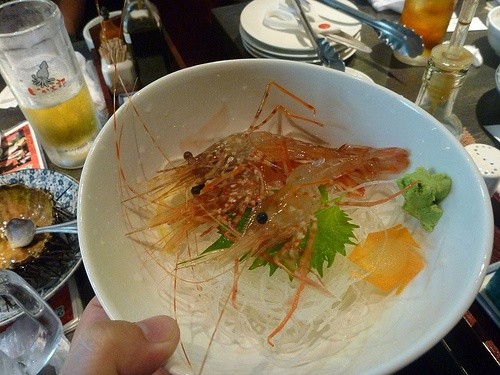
[486,5,500,55]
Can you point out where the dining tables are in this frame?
[0,0,500,375]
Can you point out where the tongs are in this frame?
[291,0,424,72]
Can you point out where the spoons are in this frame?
[5,218,78,247]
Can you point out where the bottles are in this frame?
[122,0,180,86]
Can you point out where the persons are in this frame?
[59,294,180,375]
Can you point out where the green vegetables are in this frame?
[200,184,361,282]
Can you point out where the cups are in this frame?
[394,0,457,66]
[0,0,102,168]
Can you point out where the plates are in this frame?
[0,169,82,326]
[77,58,494,375]
[240,0,362,62]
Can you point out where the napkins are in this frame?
[0,51,105,113]
[369,0,405,14]
